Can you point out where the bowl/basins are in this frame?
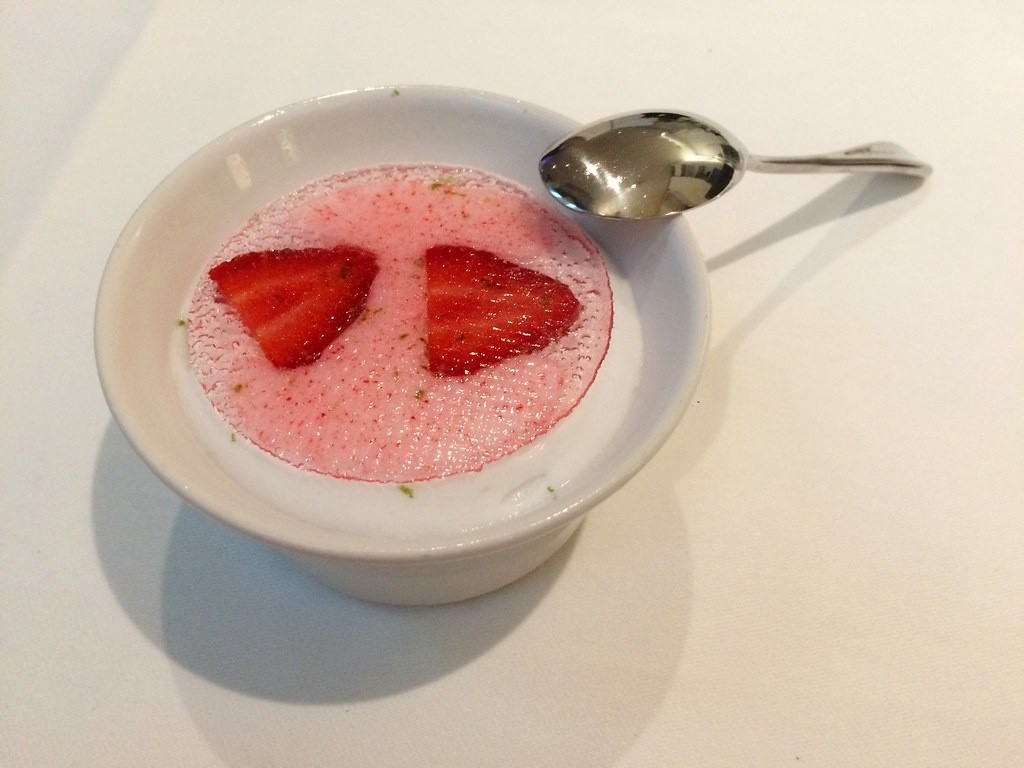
[93,83,711,566]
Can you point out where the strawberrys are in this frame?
[427,244,586,375]
[210,247,380,369]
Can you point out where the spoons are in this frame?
[538,110,931,220]
